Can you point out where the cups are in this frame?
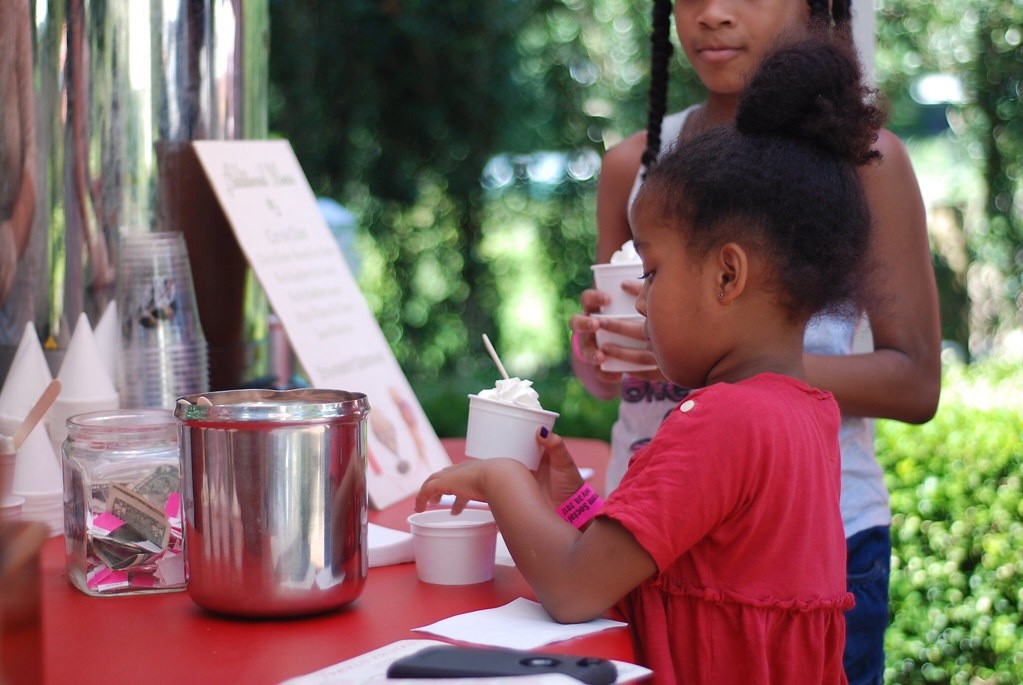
[116,227,209,412]
[587,314,659,374]
[465,394,560,474]
[589,262,645,313]
[408,507,500,585]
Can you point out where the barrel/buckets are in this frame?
[170,387,369,616]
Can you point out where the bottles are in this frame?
[64,409,186,599]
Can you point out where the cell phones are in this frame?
[386,644,618,684]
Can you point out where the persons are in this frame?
[415,32,887,684]
[570,0,943,679]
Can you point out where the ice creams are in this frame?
[591,240,644,313]
[463,376,560,472]
